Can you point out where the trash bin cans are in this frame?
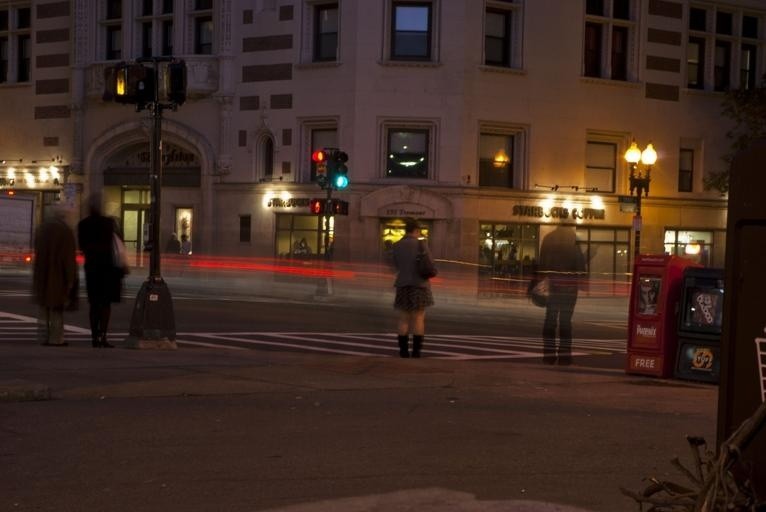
[625,253,725,384]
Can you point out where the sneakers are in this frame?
[41,343,68,347]
[543,355,571,365]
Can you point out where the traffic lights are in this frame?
[311,149,349,215]
[105,60,188,105]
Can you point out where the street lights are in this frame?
[624,137,657,255]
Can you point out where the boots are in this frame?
[397,335,424,358]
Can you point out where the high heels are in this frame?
[91,338,115,348]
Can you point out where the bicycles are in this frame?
[619,402,766,512]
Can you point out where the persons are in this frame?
[78,192,130,348]
[166,231,181,254]
[181,234,191,254]
[638,279,658,313]
[299,237,312,256]
[391,218,435,358]
[32,201,75,347]
[528,220,588,365]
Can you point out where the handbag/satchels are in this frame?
[416,252,437,278]
[110,232,130,274]
[527,278,549,307]
